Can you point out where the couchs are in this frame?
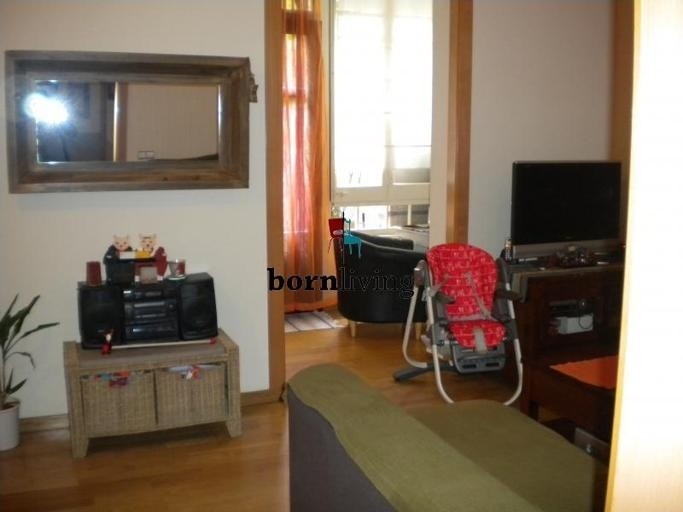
[284,360,606,511]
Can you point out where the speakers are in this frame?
[78,280,123,349]
[175,272,217,341]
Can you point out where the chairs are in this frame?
[394,243,527,409]
[327,212,428,342]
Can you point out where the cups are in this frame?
[167,259,185,277]
[96,330,113,354]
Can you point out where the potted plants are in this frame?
[0,290,59,454]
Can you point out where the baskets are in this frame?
[81,373,156,437]
[154,363,226,431]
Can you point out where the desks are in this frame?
[497,255,625,361]
[57,328,242,458]
[548,350,616,395]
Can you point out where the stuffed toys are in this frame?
[103,235,131,260]
[137,233,157,258]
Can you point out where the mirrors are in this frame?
[3,49,250,195]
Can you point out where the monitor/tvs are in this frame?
[512,162,621,271]
